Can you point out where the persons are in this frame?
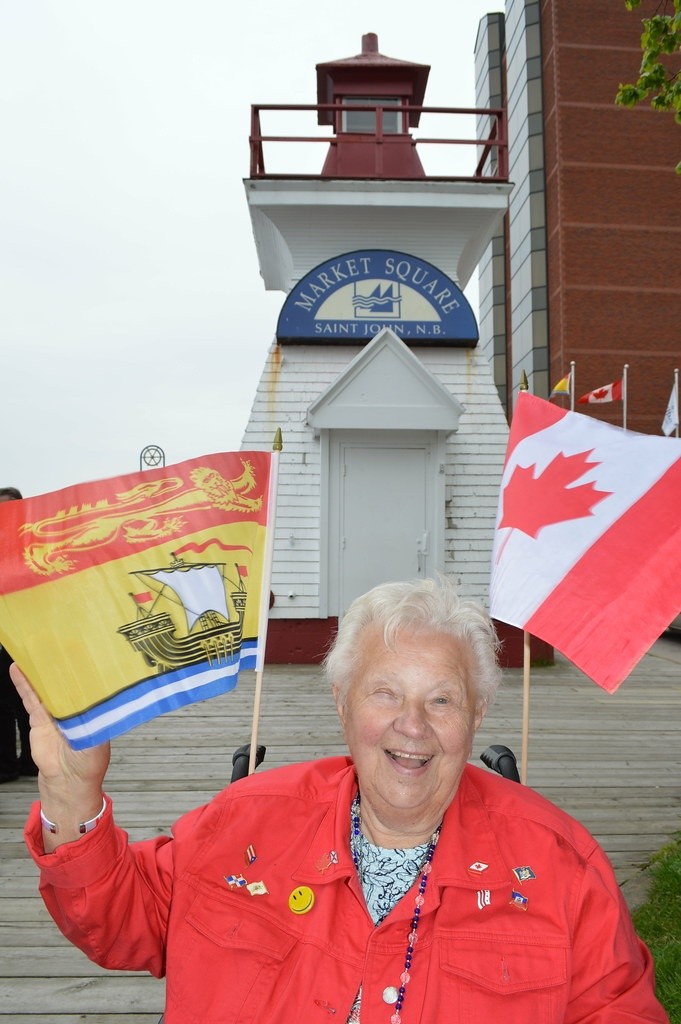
[9,577,670,1024]
[0,487,38,784]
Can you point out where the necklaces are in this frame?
[353,797,441,1024]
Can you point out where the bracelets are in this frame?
[39,798,109,833]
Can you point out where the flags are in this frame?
[547,373,570,402]
[661,377,680,436]
[1,452,271,752]
[575,379,622,404]
[490,387,680,696]
